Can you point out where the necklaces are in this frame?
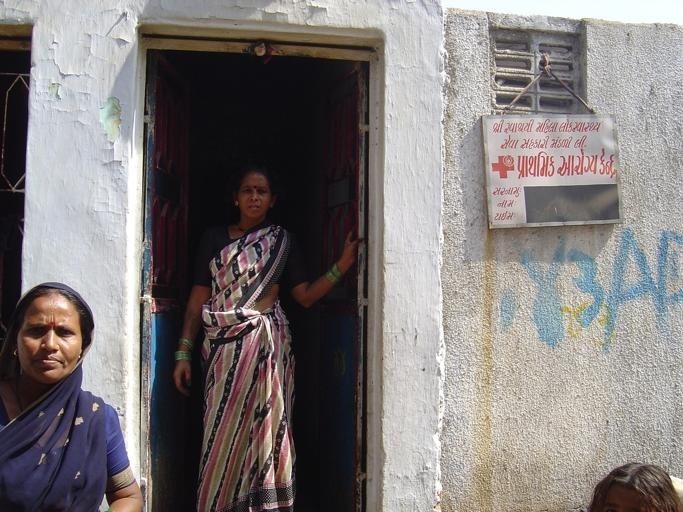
[8,376,24,413]
[234,221,245,232]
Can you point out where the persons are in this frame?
[587,461,683,512]
[173,161,365,512]
[0,282,145,512]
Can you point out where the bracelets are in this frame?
[331,263,343,281]
[179,338,195,353]
[324,269,340,286]
[174,351,193,362]
